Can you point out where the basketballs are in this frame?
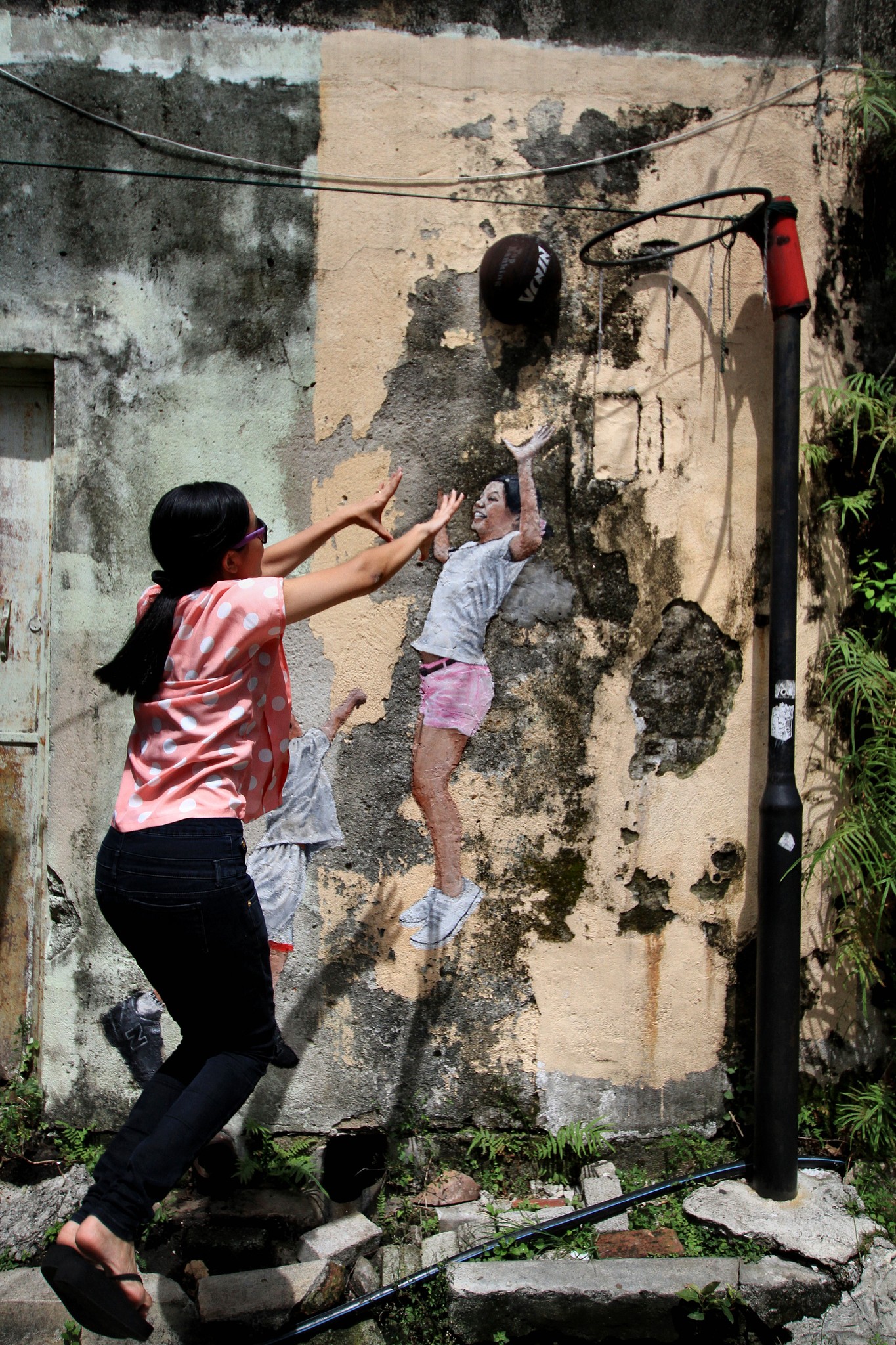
[480,234,562,325]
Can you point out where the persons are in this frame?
[36,463,464,1339]
